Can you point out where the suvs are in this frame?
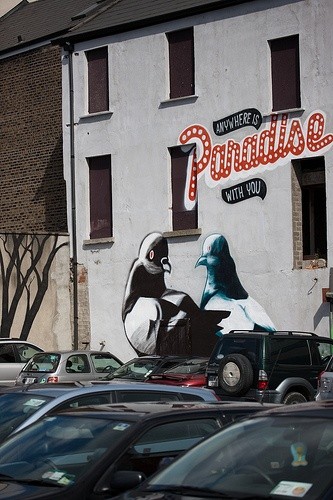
[0,338,46,388]
[204,329,333,405]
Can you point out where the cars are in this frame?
[90,355,209,386]
[316,355,332,402]
[16,350,125,383]
[0,402,287,500]
[1,385,222,447]
[109,401,332,500]
